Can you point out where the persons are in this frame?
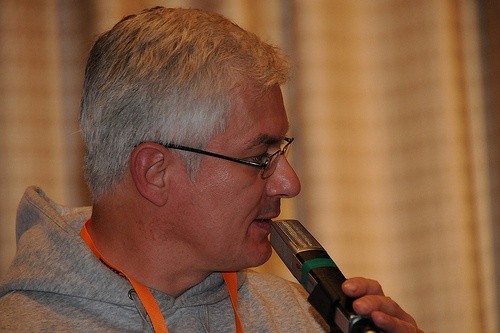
[1,4,426,333]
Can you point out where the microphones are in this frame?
[269,219,376,333]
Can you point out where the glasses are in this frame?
[159,138,295,178]
